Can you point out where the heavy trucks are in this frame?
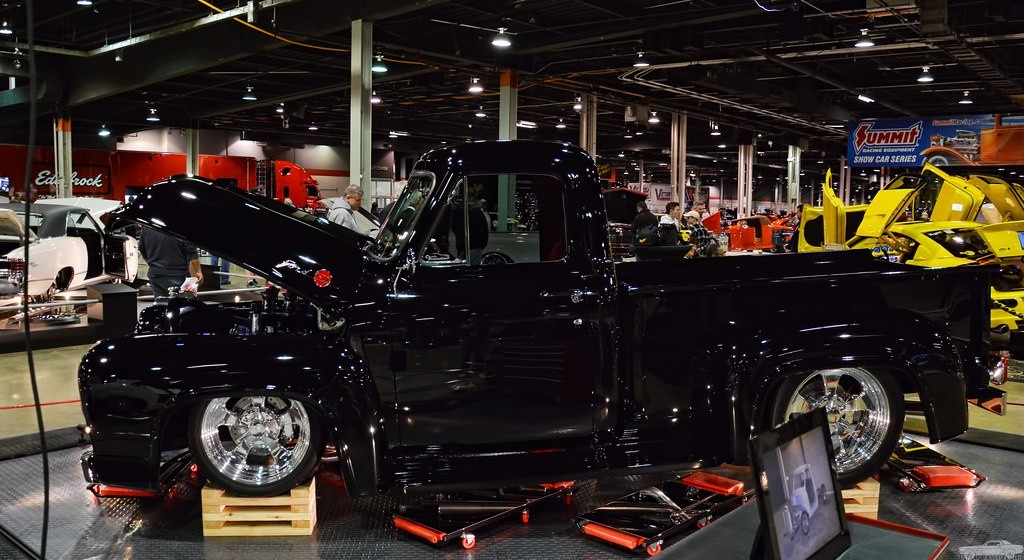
[0,143,329,218]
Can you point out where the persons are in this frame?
[630,199,710,259]
[789,204,803,254]
[245,185,265,288]
[451,186,489,266]
[210,253,232,285]
[326,184,365,233]
[137,226,204,301]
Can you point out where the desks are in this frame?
[645,495,951,560]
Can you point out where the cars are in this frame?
[0,196,140,309]
[690,160,1024,355]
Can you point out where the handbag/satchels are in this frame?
[632,224,660,245]
[659,223,678,246]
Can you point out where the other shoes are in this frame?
[221,280,231,285]
[265,283,273,287]
[247,278,261,287]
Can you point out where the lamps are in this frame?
[0,0,976,138]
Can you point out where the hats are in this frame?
[682,211,700,220]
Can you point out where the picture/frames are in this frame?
[748,407,851,560]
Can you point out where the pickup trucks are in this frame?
[78,136,1009,499]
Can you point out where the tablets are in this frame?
[748,405,852,560]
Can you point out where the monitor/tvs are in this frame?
[0,176,10,193]
